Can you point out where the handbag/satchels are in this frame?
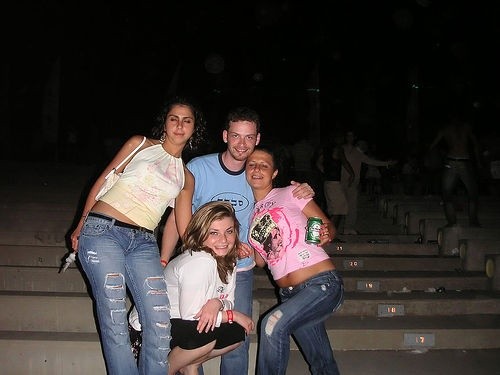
[95,136,148,201]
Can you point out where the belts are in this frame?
[89,213,153,234]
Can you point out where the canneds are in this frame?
[306,217,323,244]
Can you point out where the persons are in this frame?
[127,199,256,375]
[316,131,392,237]
[245,145,345,375]
[72,102,252,375]
[159,108,314,375]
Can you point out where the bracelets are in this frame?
[81,215,86,218]
[160,260,169,265]
[160,264,165,269]
[227,309,234,324]
